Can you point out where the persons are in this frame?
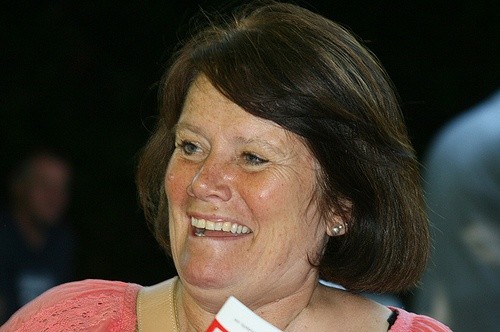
[0,152,77,327]
[0,2,453,332]
